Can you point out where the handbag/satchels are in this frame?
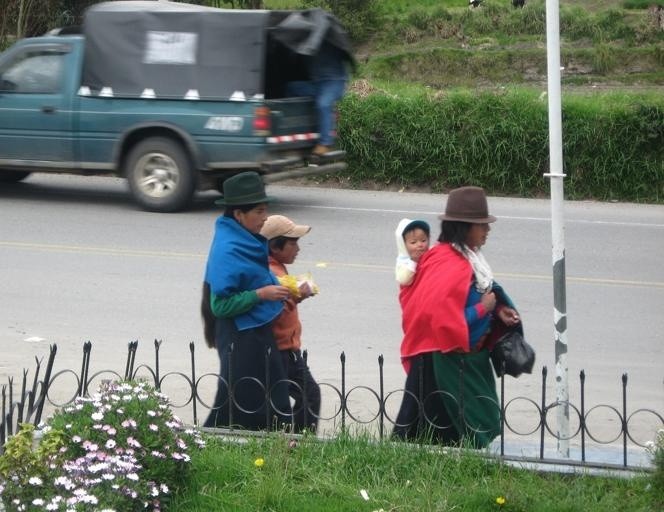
[492,329,534,377]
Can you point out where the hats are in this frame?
[438,186,496,224]
[215,172,276,206]
[259,215,311,240]
[403,220,430,234]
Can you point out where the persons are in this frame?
[276,40,351,160]
[256,214,322,436]
[389,185,521,451]
[199,170,297,432]
[393,216,431,287]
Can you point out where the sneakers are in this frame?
[313,144,335,154]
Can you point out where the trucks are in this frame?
[0,1,352,213]
